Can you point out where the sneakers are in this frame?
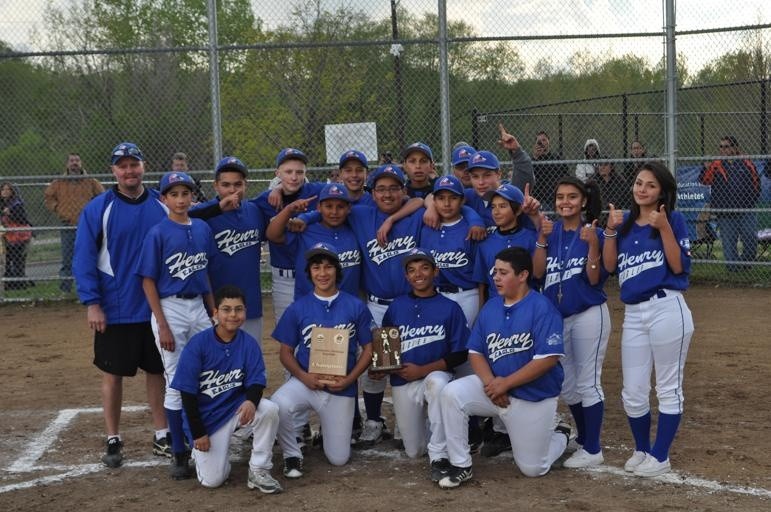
[554,420,604,468]
[430,419,512,489]
[153,416,404,494]
[102,438,124,467]
[624,449,671,477]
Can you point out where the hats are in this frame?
[159,171,196,195]
[402,247,435,269]
[319,143,525,205]
[304,243,340,262]
[276,148,308,170]
[214,157,248,180]
[112,143,143,164]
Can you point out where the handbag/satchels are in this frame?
[5,223,32,243]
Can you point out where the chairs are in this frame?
[686,205,726,258]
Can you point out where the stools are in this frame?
[755,227,771,259]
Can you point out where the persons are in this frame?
[394,350,401,366]
[44,150,106,294]
[72,122,693,478]
[380,328,393,353]
[438,244,572,490]
[369,246,473,485]
[169,285,285,496]
[696,136,762,274]
[371,351,379,368]
[1,180,37,292]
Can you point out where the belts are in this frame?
[178,293,198,298]
[643,289,665,299]
[439,285,468,293]
[280,270,297,278]
[368,295,392,306]
